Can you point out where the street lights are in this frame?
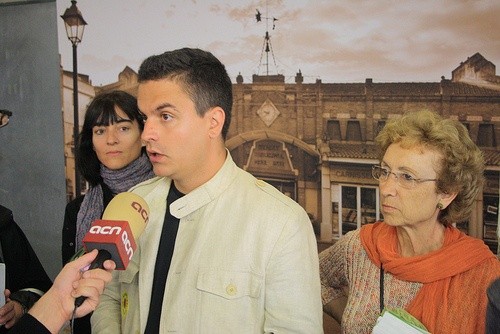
[60,0,89,198]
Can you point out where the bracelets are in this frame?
[21,304,28,314]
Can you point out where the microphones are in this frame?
[75,192,150,307]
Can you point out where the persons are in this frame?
[318,110,500,334]
[91,47,324,334]
[0,91,159,334]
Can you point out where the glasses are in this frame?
[372,164,440,188]
